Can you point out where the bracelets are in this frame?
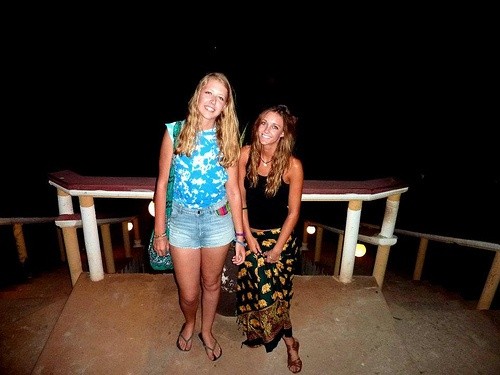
[235,233,244,236]
[269,254,279,261]
[154,233,166,238]
[242,208,248,210]
[234,239,246,247]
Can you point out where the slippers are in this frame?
[177,322,195,351]
[198,332,222,361]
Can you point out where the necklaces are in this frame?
[261,158,273,166]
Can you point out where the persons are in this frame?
[153,72,246,361]
[236,104,303,374]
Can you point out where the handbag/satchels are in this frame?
[148,227,174,270]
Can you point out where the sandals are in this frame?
[252,339,261,345]
[287,337,302,373]
